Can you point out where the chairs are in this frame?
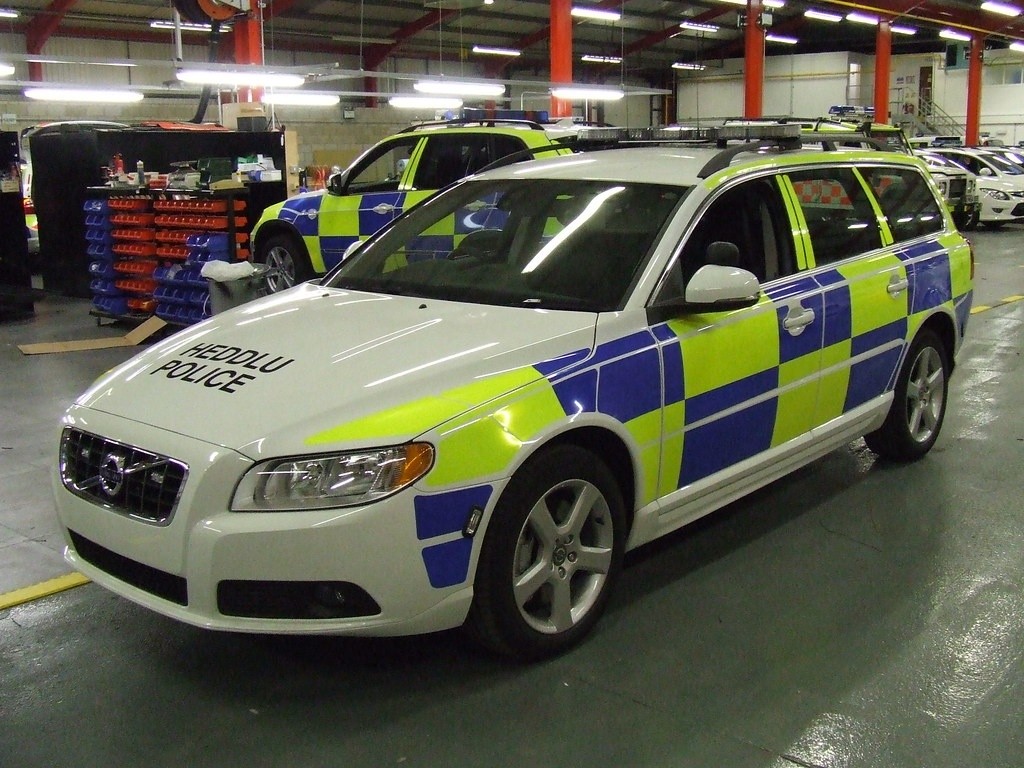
[684,201,763,281]
[425,141,465,188]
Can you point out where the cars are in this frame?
[743,106,1024,236]
[245,113,627,296]
[55,122,982,667]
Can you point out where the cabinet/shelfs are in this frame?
[82,186,254,329]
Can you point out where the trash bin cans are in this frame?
[208,262,273,317]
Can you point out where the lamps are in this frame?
[8,0,145,102]
[412,0,506,96]
[169,0,306,88]
[386,0,465,110]
[259,0,341,106]
